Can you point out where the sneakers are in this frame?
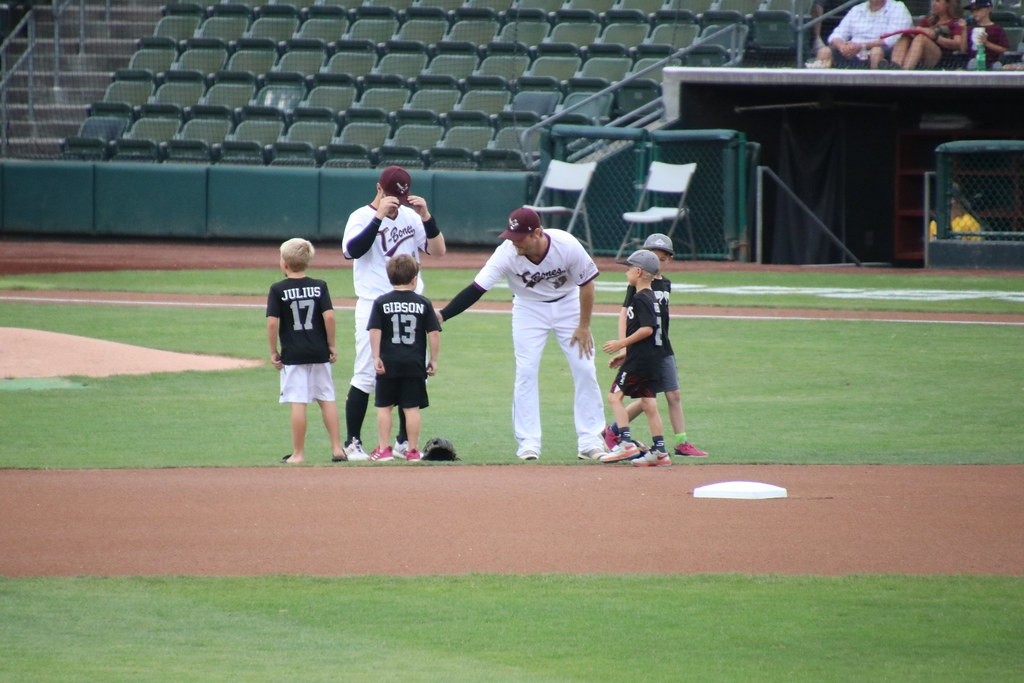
[343,437,370,462]
[393,439,424,460]
[597,426,620,453]
[577,449,608,463]
[674,441,709,458]
[404,448,421,461]
[366,446,393,462]
[600,440,640,462]
[632,449,672,467]
[519,450,539,461]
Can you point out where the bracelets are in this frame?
[422,215,440,240]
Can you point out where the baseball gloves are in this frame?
[421,437,462,460]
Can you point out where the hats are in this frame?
[379,165,412,205]
[642,233,674,255]
[497,207,541,242]
[421,437,457,461]
[616,250,660,275]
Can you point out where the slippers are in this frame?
[332,448,348,461]
[279,454,292,463]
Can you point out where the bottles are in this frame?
[977,43,986,70]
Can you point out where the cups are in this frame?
[972,26,985,50]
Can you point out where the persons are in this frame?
[804,0,913,70]
[877,0,962,70]
[342,166,446,466]
[265,238,347,464]
[929,181,981,241]
[599,233,708,468]
[365,254,442,462]
[437,207,608,459]
[958,0,1009,71]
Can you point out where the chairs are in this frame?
[522,160,598,256]
[616,160,697,262]
[64,0,1024,176]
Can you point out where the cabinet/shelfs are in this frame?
[893,124,1024,259]
